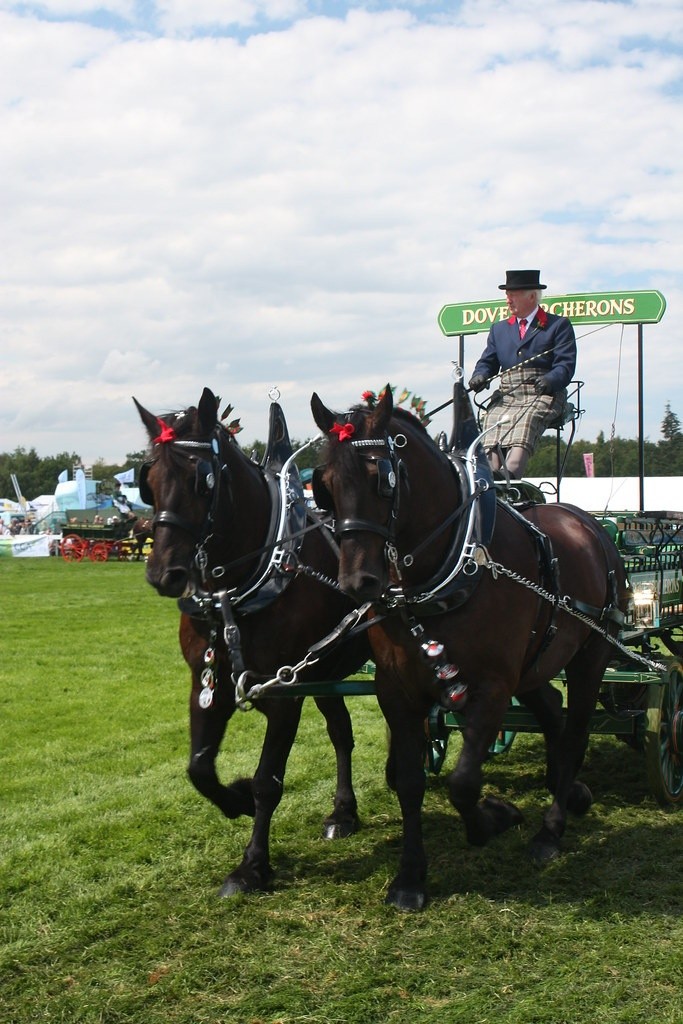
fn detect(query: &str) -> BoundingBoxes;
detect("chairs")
[474,380,588,504]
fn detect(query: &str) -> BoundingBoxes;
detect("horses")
[309,390,632,915]
[132,387,370,903]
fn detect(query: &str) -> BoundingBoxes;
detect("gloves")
[469,374,490,392]
[528,376,552,396]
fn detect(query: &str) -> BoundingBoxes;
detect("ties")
[519,319,528,339]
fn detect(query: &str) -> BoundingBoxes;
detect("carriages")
[60,508,152,562]
[131,380,681,911]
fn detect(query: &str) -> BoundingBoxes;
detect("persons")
[468,269,576,480]
[0,516,34,536]
[111,483,130,516]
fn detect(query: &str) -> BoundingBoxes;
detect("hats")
[498,270,547,289]
